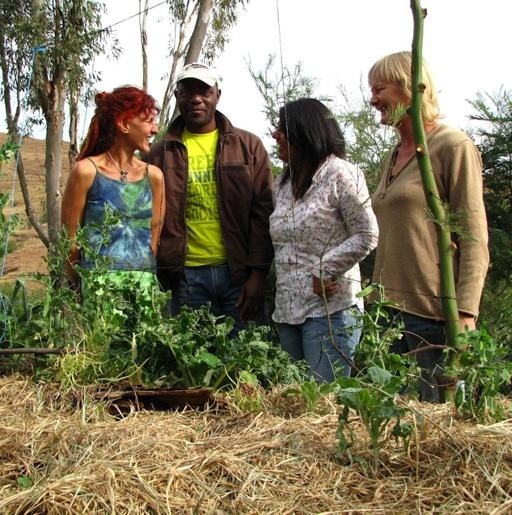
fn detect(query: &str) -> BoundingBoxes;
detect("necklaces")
[107,148,134,184]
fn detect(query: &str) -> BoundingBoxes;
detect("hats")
[170,61,220,88]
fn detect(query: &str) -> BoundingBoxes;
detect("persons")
[60,83,168,319]
[264,96,382,384]
[140,61,275,344]
[366,45,492,406]
